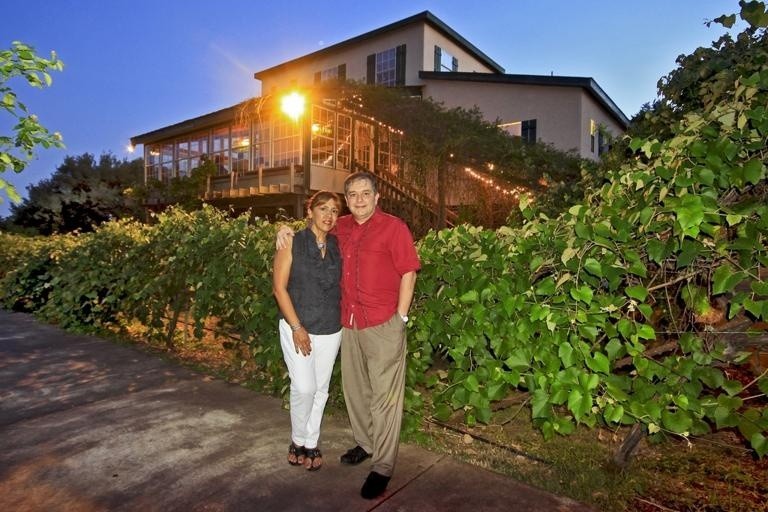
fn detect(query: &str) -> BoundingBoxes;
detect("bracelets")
[290,321,302,332]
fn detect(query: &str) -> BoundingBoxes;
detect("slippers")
[288,442,306,465]
[304,449,323,470]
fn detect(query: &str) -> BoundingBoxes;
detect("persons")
[275,172,421,499]
[272,190,344,472]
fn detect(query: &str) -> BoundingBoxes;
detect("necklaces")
[316,237,326,250]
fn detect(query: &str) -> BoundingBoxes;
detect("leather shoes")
[340,446,372,465]
[361,471,391,499]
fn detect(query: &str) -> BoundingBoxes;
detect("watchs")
[400,315,409,323]
